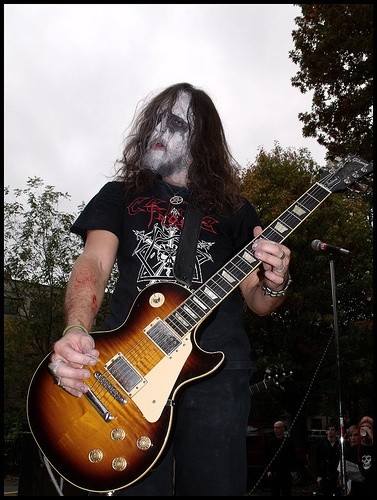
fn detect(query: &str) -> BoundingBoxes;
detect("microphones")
[311,239,350,255]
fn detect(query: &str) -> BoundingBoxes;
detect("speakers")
[17,431,80,496]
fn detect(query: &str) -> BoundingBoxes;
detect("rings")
[56,378,62,386]
[279,251,286,261]
[272,265,285,274]
[48,360,63,378]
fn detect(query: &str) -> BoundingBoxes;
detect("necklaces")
[161,177,186,205]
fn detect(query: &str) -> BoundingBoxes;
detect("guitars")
[27,151,374,493]
[250,366,293,399]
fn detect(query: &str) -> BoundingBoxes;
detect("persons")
[48,83,290,500]
[265,417,373,498]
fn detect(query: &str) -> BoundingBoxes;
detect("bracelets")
[63,322,89,336]
[262,274,292,297]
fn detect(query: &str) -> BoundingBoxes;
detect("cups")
[360,430,368,436]
